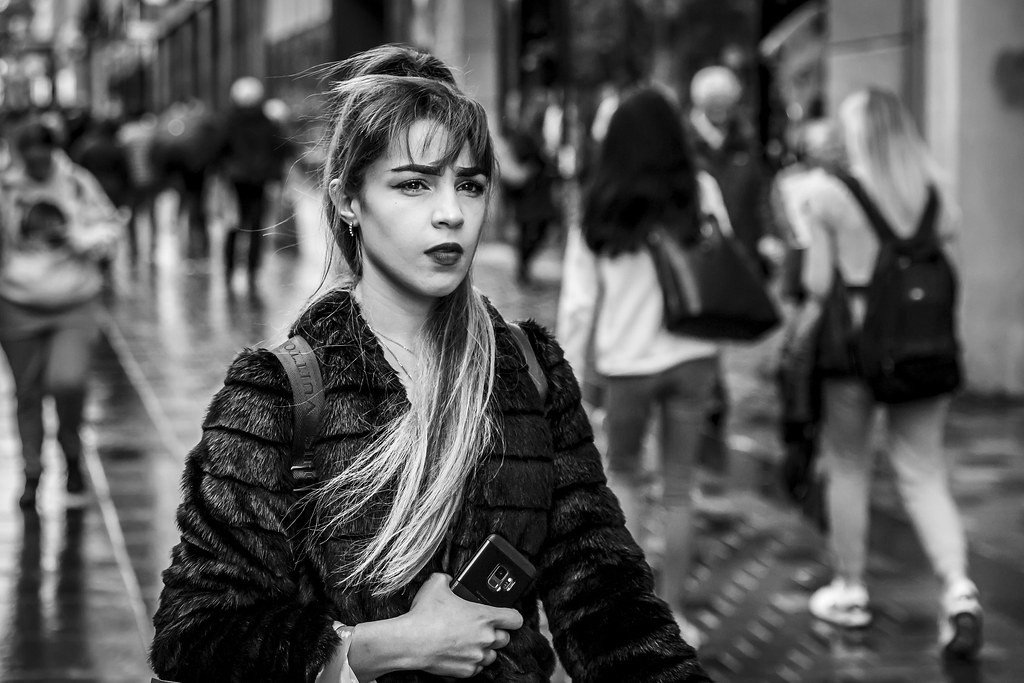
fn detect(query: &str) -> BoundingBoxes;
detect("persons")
[150,45,713,683]
[499,58,986,666]
[2,68,301,509]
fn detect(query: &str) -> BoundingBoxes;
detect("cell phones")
[449,532,540,608]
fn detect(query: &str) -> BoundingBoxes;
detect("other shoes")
[809,581,872,627]
[19,474,40,509]
[66,455,84,493]
[938,586,985,668]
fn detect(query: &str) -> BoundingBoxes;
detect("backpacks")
[823,169,964,408]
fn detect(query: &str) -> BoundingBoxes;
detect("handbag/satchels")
[646,213,784,344]
